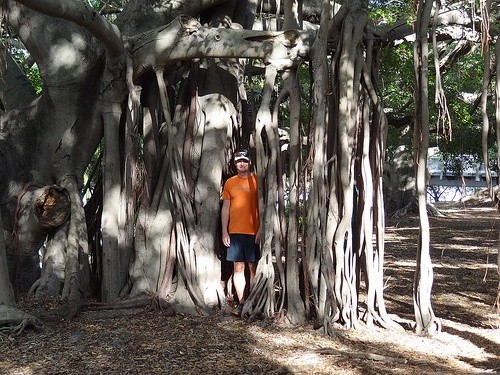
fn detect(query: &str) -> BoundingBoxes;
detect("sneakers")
[232,304,243,316]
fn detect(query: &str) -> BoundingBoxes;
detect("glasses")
[234,153,250,157]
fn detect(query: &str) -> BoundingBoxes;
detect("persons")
[220,147,263,317]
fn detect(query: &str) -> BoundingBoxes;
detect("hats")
[234,148,251,161]
